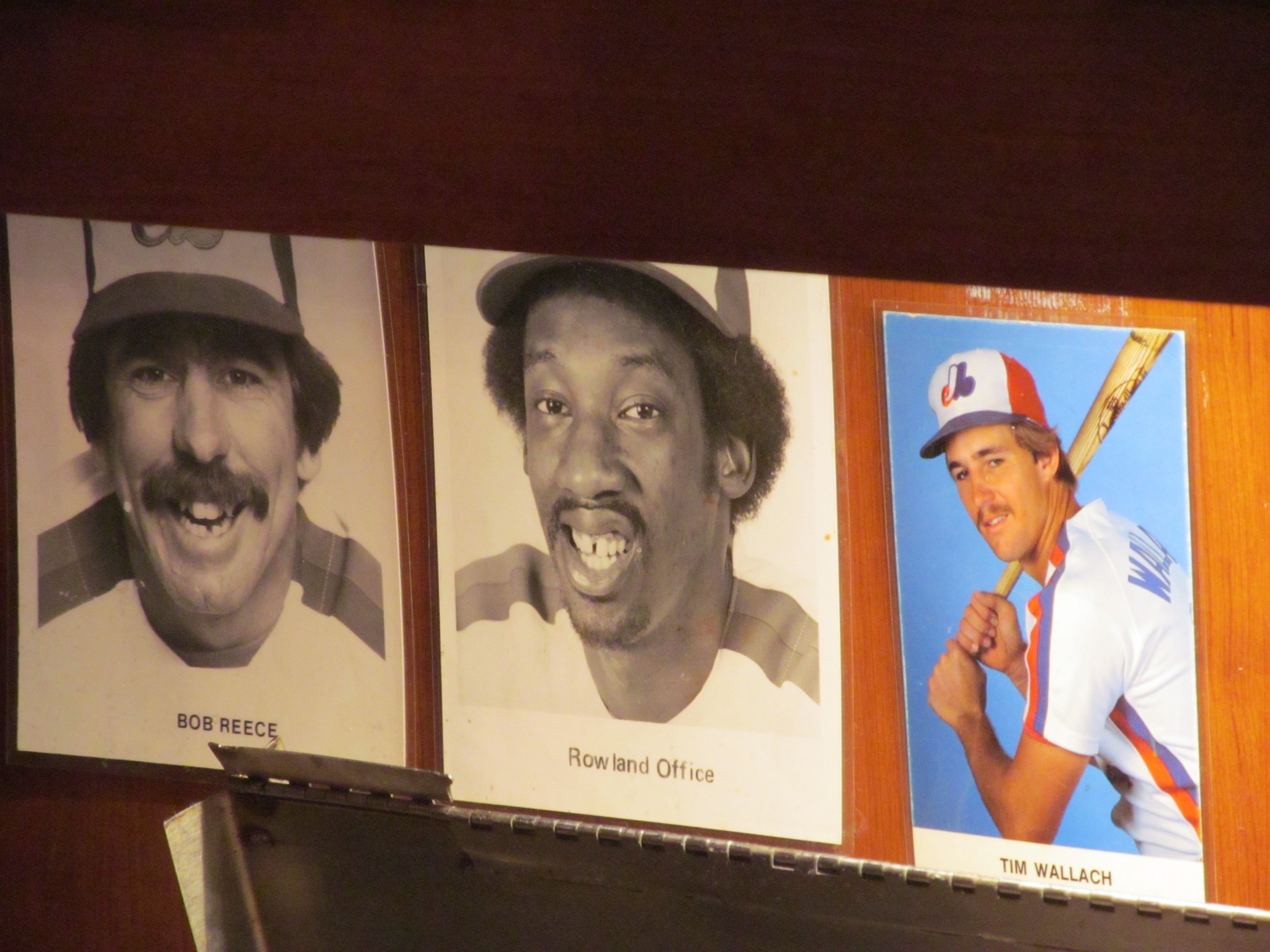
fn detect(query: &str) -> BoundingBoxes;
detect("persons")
[920,348,1203,859]
[36,220,386,670]
[453,253,822,728]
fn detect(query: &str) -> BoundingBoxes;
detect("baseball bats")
[995,330,1175,600]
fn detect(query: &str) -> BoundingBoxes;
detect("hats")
[920,348,1048,459]
[72,219,303,340]
[475,248,752,338]
